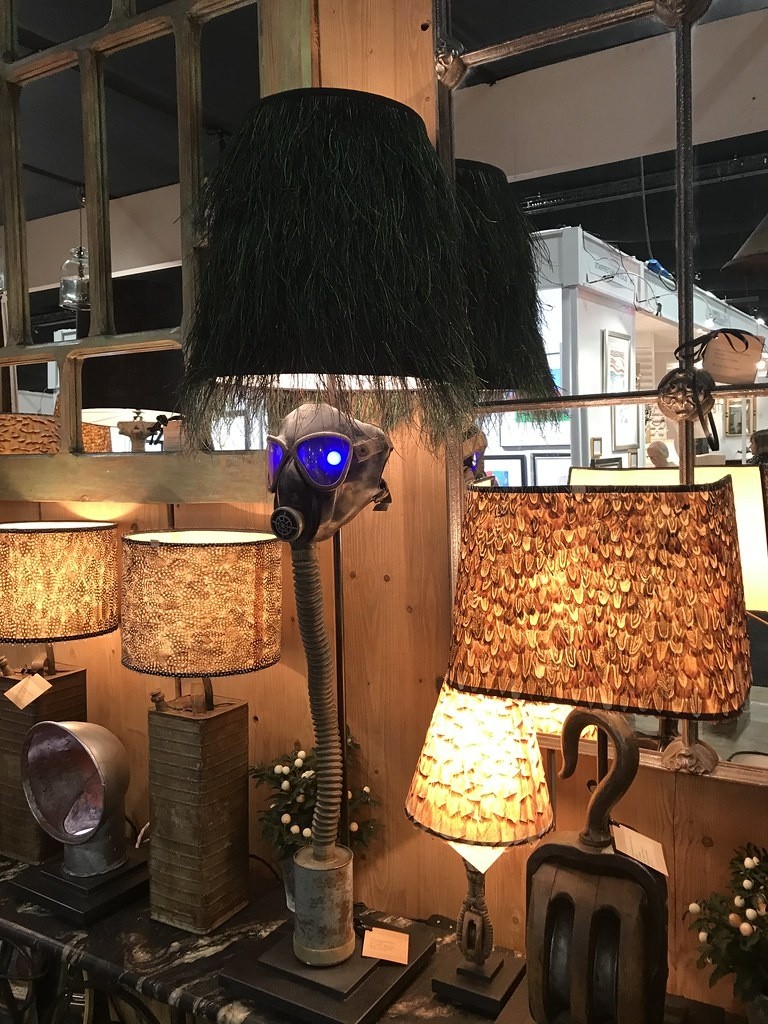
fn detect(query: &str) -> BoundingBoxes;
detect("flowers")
[682,841,768,1005]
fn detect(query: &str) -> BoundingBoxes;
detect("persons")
[646,441,678,467]
[747,429,768,509]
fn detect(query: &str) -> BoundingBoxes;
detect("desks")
[634,686,768,763]
[0,855,748,1024]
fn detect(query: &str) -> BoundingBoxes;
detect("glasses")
[262,434,384,492]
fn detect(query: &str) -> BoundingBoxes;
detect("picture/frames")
[499,352,570,448]
[602,329,632,394]
[590,437,603,458]
[628,449,638,468]
[595,457,622,468]
[611,405,640,452]
[483,455,527,486]
[211,410,251,450]
[530,452,572,486]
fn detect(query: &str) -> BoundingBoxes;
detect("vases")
[283,857,295,913]
[746,994,768,1024]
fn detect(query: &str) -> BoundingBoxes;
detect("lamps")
[179,87,495,1024]
[0,519,120,867]
[0,412,61,455]
[566,464,768,752]
[603,272,662,317]
[120,527,284,935]
[446,475,753,1024]
[521,701,597,741]
[404,672,555,1019]
[660,291,714,327]
[453,157,568,424]
[82,422,112,452]
